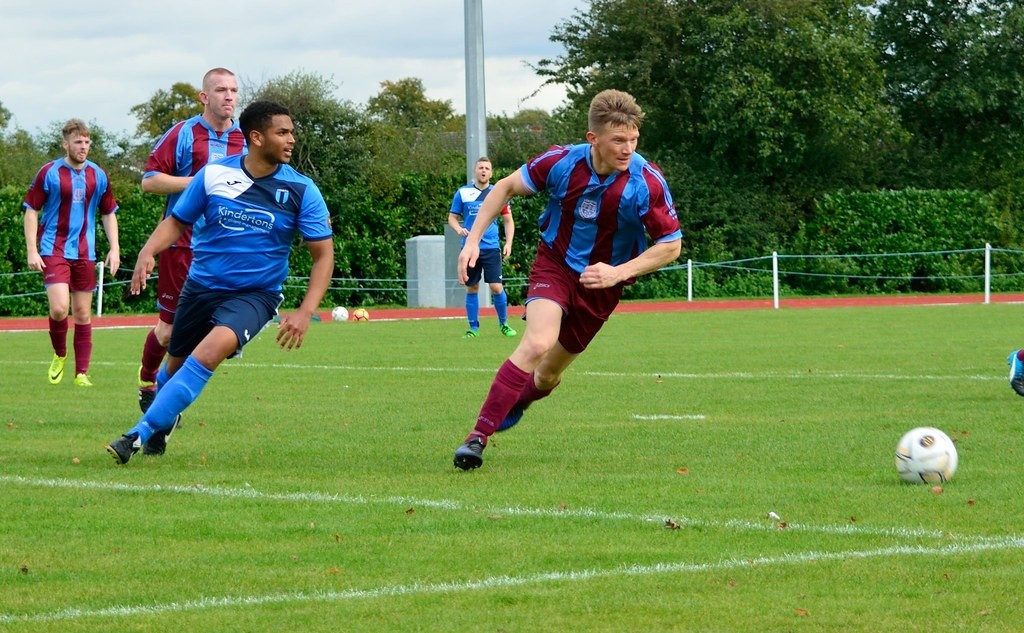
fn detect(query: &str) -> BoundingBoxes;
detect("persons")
[448,158,517,339]
[22,120,120,386]
[1007,349,1024,397]
[453,88,681,470]
[105,102,336,466]
[136,69,251,411]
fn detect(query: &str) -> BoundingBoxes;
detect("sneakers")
[48,352,67,384]
[106,431,142,464]
[499,323,518,337]
[138,387,158,416]
[453,445,484,471]
[73,373,93,389]
[1007,348,1024,396]
[462,329,479,340]
[494,406,524,432]
[143,412,182,455]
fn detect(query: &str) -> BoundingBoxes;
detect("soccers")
[895,426,958,483]
[332,306,349,321]
[352,309,370,321]
